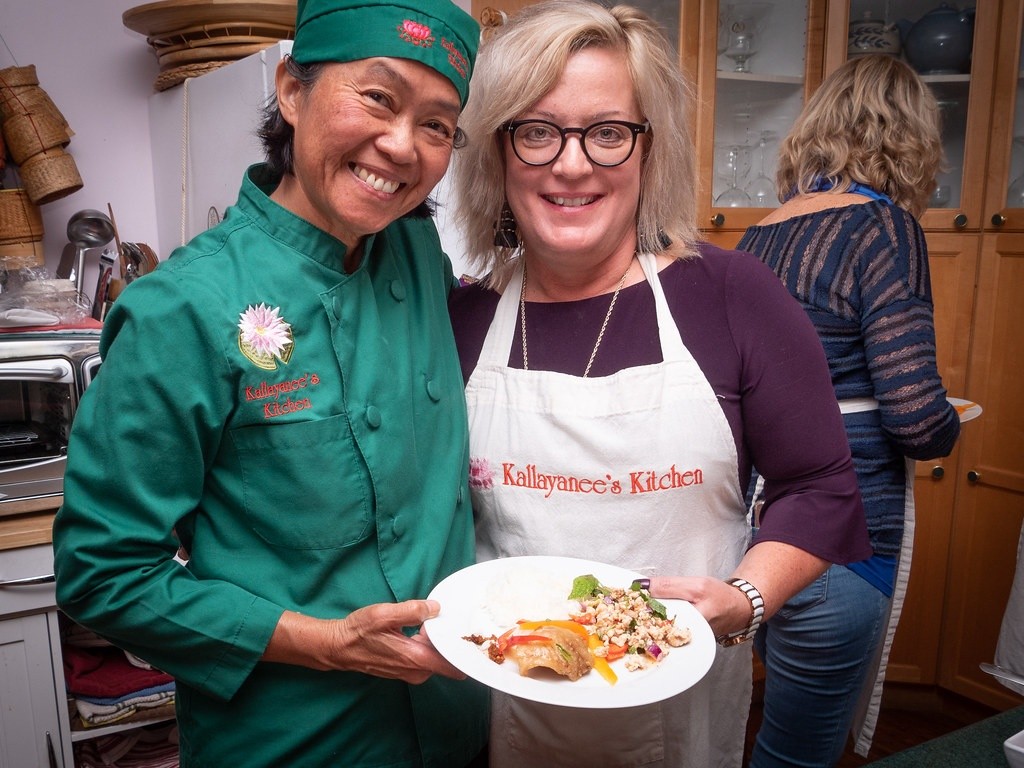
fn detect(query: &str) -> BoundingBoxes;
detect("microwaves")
[0,334,102,499]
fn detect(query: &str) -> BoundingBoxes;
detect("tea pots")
[849,4,975,73]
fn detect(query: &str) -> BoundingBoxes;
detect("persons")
[441,0,873,768]
[726,59,961,768]
[48,0,494,768]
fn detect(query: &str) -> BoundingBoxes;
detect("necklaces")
[521,260,630,377]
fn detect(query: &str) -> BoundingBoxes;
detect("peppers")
[497,621,618,684]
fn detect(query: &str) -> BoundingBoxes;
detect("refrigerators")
[146,39,527,288]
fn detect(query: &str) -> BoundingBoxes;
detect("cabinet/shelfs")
[469,0,1024,710]
[0,532,189,768]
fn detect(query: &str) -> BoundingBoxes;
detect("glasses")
[498,119,650,167]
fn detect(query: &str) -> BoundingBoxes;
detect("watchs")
[715,579,765,648]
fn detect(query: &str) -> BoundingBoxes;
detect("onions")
[579,596,664,662]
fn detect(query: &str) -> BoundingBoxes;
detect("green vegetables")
[567,573,669,654]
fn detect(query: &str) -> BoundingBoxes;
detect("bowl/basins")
[1004,730,1024,768]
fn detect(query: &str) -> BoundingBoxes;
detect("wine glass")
[1007,137,1024,208]
[712,128,777,208]
[716,13,762,74]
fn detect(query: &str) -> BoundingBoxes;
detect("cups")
[929,186,951,208]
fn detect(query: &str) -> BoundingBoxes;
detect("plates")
[424,556,716,709]
[946,397,983,423]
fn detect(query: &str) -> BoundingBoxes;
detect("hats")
[292,0,480,112]
